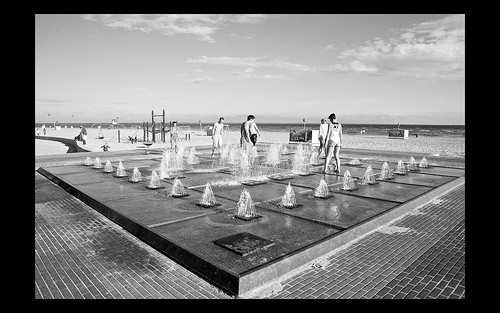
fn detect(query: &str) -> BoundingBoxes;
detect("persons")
[170,120,179,141]
[43,128,46,135]
[80,126,87,145]
[249,122,261,146]
[211,117,224,156]
[322,113,342,174]
[240,115,255,146]
[36,129,39,135]
[319,119,328,156]
[101,142,110,152]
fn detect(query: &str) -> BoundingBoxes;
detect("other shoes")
[321,171,327,174]
[333,169,340,172]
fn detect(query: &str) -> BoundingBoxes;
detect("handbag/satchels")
[250,134,258,143]
